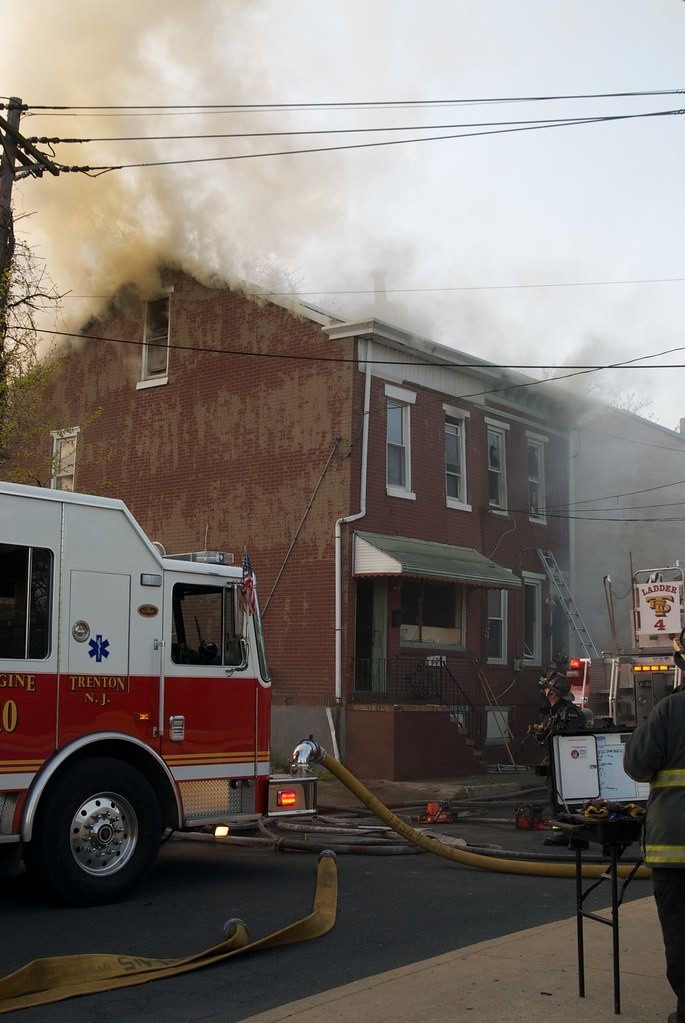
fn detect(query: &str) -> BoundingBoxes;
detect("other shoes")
[668,1012,677,1023]
[567,837,589,851]
[543,831,568,845]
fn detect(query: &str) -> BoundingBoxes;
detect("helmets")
[545,672,575,701]
[673,626,685,672]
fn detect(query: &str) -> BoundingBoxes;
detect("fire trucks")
[535,551,685,821]
[1,479,655,907]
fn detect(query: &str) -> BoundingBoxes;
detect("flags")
[241,551,255,615]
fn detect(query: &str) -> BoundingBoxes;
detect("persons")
[528,672,590,850]
[623,624,685,1022]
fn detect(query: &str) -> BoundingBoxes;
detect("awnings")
[351,531,521,590]
